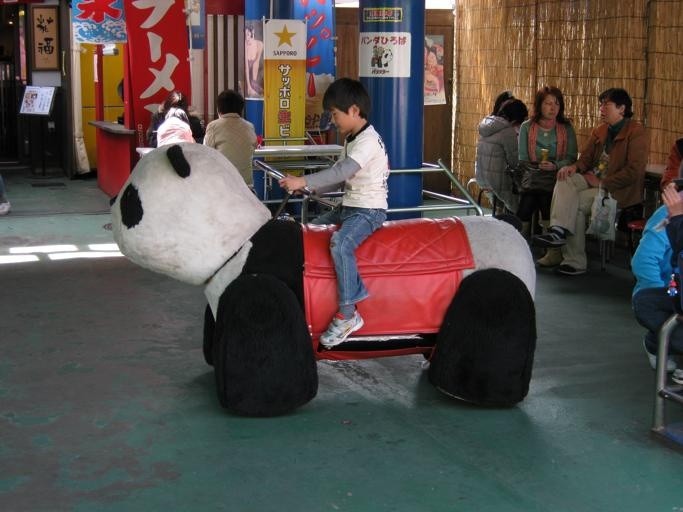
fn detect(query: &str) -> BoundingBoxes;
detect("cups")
[540,148,550,163]
[672,179,683,193]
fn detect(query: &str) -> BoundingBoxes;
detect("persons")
[0,174,11,215]
[244,26,265,99]
[146,88,258,194]
[279,79,391,350]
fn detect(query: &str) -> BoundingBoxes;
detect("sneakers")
[556,264,587,276]
[536,255,558,268]
[536,230,568,247]
[320,308,364,347]
[0,201,11,215]
[642,339,677,373]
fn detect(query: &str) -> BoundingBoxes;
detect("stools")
[477,189,509,218]
[598,203,642,266]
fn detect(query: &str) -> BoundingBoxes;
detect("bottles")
[667,273,678,296]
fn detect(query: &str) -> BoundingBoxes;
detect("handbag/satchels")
[508,159,556,197]
[586,178,617,242]
